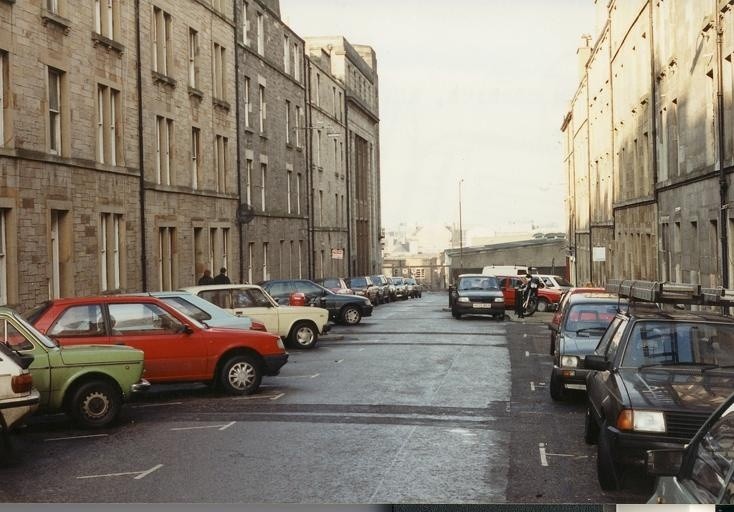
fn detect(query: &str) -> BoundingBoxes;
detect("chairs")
[97,315,122,337]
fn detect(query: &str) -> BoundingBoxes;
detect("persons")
[196,270,214,285]
[209,267,231,285]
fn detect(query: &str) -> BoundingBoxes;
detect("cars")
[2,306,151,430]
[580,312,733,493]
[554,288,607,318]
[179,283,331,350]
[317,274,422,302]
[451,264,572,320]
[64,291,251,331]
[0,342,42,459]
[552,292,665,397]
[639,396,734,504]
[4,294,291,397]
[256,281,373,327]
